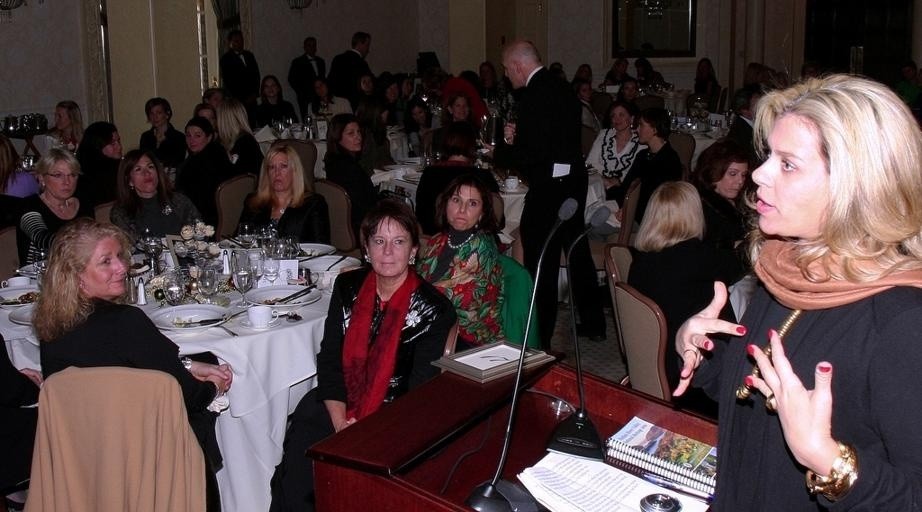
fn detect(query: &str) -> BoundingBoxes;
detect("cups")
[237,223,256,256]
[197,269,218,298]
[32,248,48,277]
[163,278,186,305]
[263,257,280,286]
[279,236,297,259]
[247,258,262,285]
[261,231,279,260]
[1,278,32,289]
[144,236,162,272]
[248,306,279,327]
[298,268,320,286]
[232,267,252,309]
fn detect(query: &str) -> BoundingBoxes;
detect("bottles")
[230,251,239,274]
[128,277,136,304]
[137,278,147,305]
[222,250,230,274]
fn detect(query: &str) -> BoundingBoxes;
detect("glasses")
[41,172,79,181]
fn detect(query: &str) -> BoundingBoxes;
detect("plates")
[0,285,41,308]
[299,255,362,277]
[148,303,233,333]
[8,305,35,326]
[18,264,36,279]
[243,284,323,310]
[299,243,338,257]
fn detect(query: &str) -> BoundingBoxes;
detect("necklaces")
[444,228,478,247]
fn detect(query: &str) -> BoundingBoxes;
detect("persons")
[16,147,88,266]
[34,219,232,474]
[219,100,263,170]
[139,96,188,171]
[630,183,736,376]
[414,139,506,234]
[427,92,486,163]
[615,77,638,103]
[385,78,399,123]
[581,84,603,132]
[178,118,234,228]
[383,81,401,125]
[687,144,751,278]
[686,59,720,114]
[223,33,261,117]
[234,143,329,242]
[258,76,299,127]
[353,73,385,125]
[722,84,763,152]
[571,63,591,97]
[599,58,633,83]
[549,61,566,86]
[0,335,42,500]
[672,75,922,511]
[500,42,606,347]
[0,130,40,220]
[397,80,411,114]
[193,103,219,139]
[585,104,647,189]
[307,76,352,131]
[270,202,455,511]
[327,33,374,101]
[203,89,222,104]
[404,97,440,154]
[476,63,501,99]
[51,100,84,153]
[476,60,499,98]
[411,178,505,351]
[586,107,682,243]
[325,113,390,246]
[110,149,204,243]
[71,121,127,216]
[636,58,667,94]
[289,36,327,123]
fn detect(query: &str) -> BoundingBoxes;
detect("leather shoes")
[573,322,605,341]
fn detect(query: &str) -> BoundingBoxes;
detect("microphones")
[466,198,579,512]
[547,205,611,459]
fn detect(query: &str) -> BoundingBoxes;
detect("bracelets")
[804,444,860,504]
[178,354,193,377]
[203,378,222,396]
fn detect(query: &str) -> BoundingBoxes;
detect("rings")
[765,395,775,412]
[684,348,699,356]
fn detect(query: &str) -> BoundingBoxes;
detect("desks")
[303,340,721,512]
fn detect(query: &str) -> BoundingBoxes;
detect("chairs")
[716,87,729,115]
[602,242,634,360]
[35,365,191,512]
[581,123,599,160]
[668,129,695,184]
[313,178,361,257]
[612,280,671,401]
[376,189,414,213]
[95,201,113,226]
[215,171,256,243]
[498,253,538,349]
[440,318,461,374]
[433,192,506,236]
[0,221,21,288]
[584,179,644,264]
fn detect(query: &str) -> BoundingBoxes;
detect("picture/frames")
[429,338,557,383]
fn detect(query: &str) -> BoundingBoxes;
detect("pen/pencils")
[639,468,715,505]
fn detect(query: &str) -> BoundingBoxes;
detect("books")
[515,453,709,512]
[608,416,718,496]
